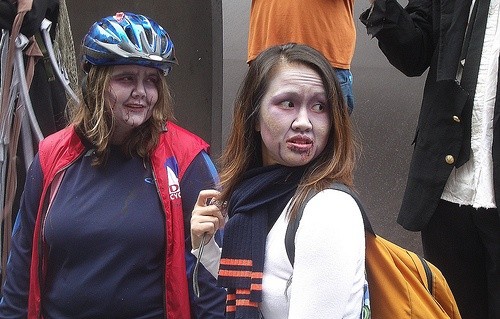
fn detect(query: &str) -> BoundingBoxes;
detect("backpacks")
[284,180,461,319]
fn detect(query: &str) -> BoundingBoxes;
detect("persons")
[246,0,357,116]
[359,0,500,319]
[190,42,372,319]
[0,11,229,319]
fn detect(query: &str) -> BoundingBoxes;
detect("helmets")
[81,12,179,75]
